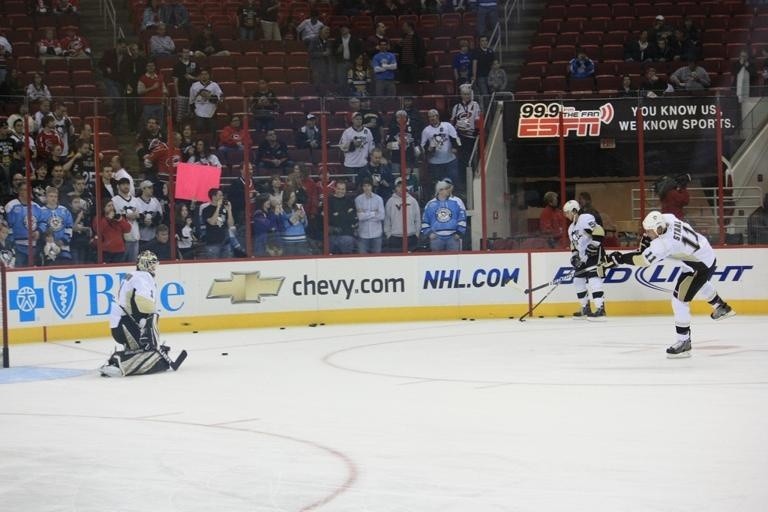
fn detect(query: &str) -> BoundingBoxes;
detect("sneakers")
[666,339,692,354]
[710,302,731,320]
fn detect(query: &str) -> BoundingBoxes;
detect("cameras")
[222,200,228,205]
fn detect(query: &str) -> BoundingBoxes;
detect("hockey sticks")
[505,261,617,294]
[519,284,560,322]
[113,299,187,370]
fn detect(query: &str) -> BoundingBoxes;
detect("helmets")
[136,249,159,276]
[563,200,580,213]
[642,211,666,230]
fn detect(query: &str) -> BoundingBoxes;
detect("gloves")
[574,261,585,271]
[570,254,580,265]
[606,251,624,267]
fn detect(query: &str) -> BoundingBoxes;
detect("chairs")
[514,1,768,99]
[509,230,630,251]
[0,0,509,253]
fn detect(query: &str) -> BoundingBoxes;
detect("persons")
[539,191,603,249]
[619,10,758,95]
[561,199,607,322]
[607,210,736,354]
[1,0,498,267]
[658,175,690,220]
[99,250,172,378]
[569,51,595,78]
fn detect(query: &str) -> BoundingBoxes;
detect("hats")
[396,110,408,116]
[428,109,439,116]
[364,113,378,119]
[141,180,154,191]
[394,177,403,185]
[351,112,362,119]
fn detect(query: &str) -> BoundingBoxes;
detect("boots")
[587,302,606,317]
[574,300,591,316]
